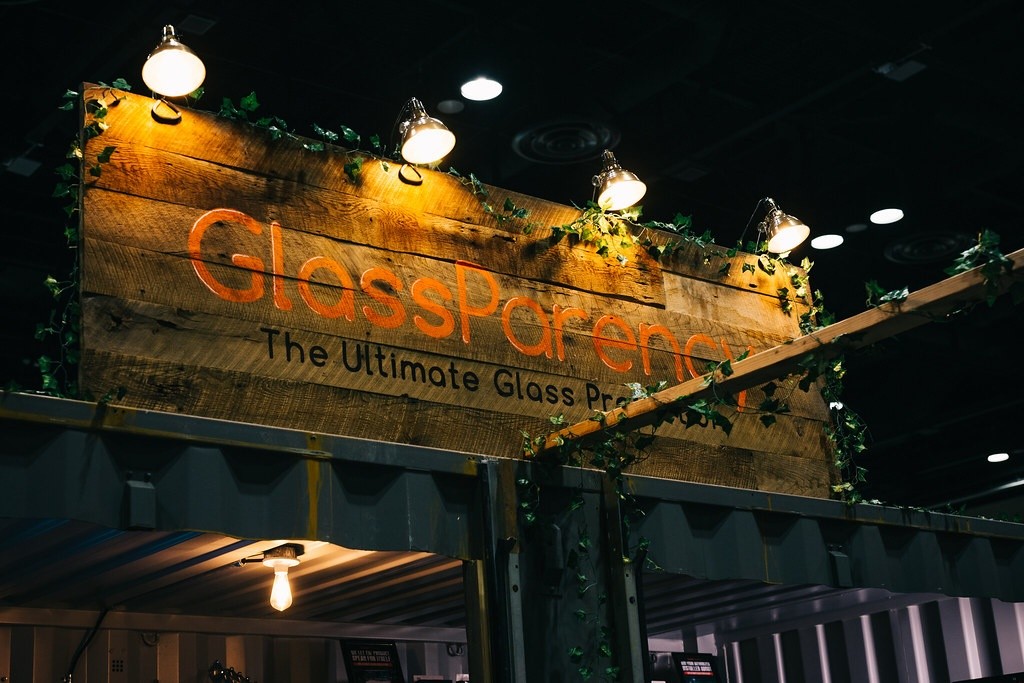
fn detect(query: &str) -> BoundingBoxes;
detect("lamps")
[398,97,455,186]
[261,548,302,613]
[588,150,647,234]
[739,197,812,275]
[140,24,210,122]
